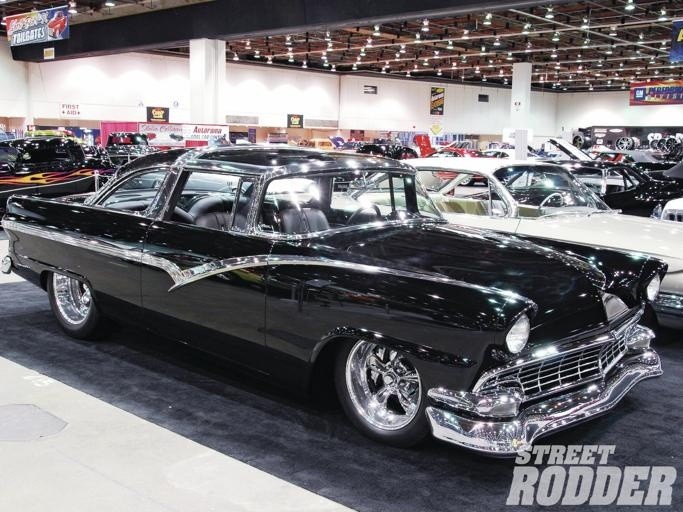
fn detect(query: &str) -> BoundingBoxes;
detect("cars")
[0,138,683,459]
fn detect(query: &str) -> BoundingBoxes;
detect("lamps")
[188,192,331,236]
[431,193,515,215]
